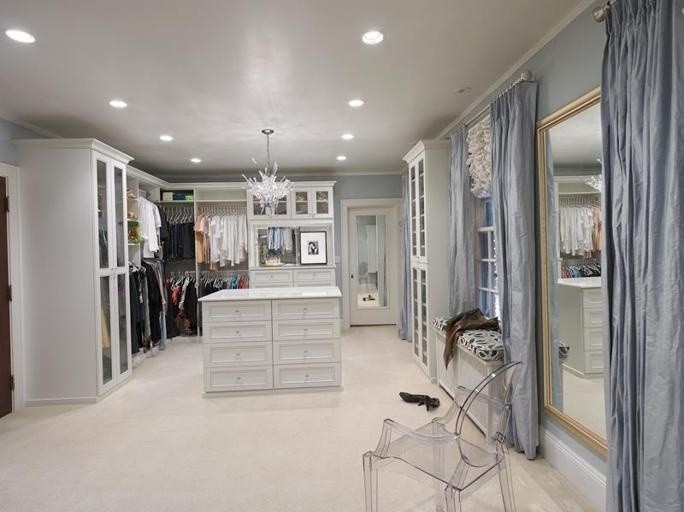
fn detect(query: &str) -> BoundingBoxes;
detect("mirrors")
[353,214,390,311]
[534,85,609,464]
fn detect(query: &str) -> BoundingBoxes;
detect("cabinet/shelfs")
[167,182,335,343]
[557,276,605,380]
[402,138,452,379]
[202,298,342,393]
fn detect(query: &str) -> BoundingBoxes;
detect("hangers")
[557,195,602,270]
[160,203,246,226]
[128,255,250,288]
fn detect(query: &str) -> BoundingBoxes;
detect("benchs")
[432,313,505,443]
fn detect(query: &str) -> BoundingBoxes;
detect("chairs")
[358,262,369,293]
[363,361,526,512]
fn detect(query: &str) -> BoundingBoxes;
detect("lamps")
[240,128,294,218]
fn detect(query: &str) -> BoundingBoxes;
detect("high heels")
[399,392,439,411]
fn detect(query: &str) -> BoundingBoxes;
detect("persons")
[309,242,318,254]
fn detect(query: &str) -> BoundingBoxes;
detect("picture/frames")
[299,231,328,266]
